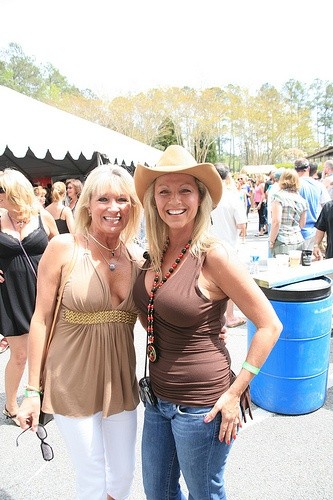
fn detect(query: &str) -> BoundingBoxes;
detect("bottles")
[302,249,312,266]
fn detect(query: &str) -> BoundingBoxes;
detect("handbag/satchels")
[139,376,156,406]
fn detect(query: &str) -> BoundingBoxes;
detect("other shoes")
[239,225,267,238]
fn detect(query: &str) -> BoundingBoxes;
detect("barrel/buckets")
[248,272,333,416]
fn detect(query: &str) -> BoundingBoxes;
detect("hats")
[134,144,222,210]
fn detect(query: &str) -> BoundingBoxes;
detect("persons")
[17,164,228,500]
[33,186,47,206]
[44,181,76,234]
[231,158,333,238]
[132,144,283,500]
[293,157,332,251]
[211,162,248,329]
[313,199,333,262]
[0,167,61,427]
[0,337,10,353]
[64,179,84,219]
[268,168,309,262]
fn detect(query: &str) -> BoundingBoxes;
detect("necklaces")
[85,226,121,256]
[91,238,122,271]
[146,236,193,363]
[8,211,27,230]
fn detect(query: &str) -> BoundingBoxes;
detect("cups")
[289,250,302,266]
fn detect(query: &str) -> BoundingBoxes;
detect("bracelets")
[270,240,275,245]
[242,361,260,376]
[314,244,319,248]
[23,385,44,398]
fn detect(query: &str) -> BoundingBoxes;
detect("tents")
[0,84,165,183]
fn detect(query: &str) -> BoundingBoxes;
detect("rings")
[233,417,240,425]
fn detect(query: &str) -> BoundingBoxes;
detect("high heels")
[2,404,20,427]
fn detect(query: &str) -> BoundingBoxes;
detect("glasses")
[237,178,243,180]
[16,423,54,462]
[0,187,6,194]
